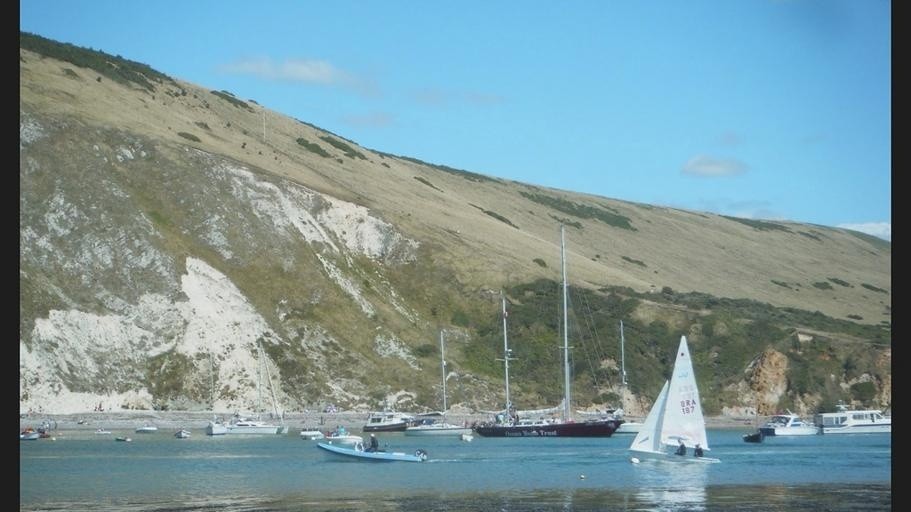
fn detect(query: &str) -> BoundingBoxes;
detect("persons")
[363,434,379,453]
[673,438,686,456]
[693,443,703,458]
[335,425,339,436]
[339,425,345,435]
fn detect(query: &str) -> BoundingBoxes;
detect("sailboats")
[629,335,721,467]
[227,342,289,433]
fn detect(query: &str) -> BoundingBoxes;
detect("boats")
[20,426,190,442]
[744,410,891,443]
[299,430,428,463]
[206,422,225,435]
